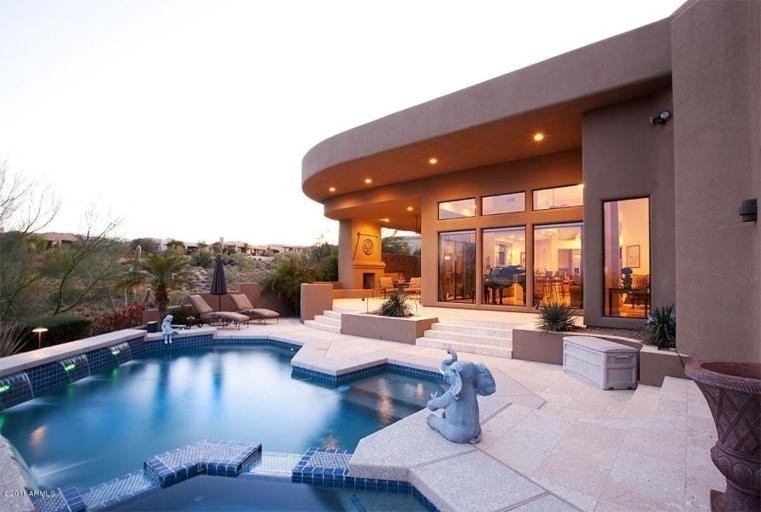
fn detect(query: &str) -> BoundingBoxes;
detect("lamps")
[31,325,48,349]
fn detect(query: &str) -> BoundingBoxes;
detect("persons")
[160,314,174,345]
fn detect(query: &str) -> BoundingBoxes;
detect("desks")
[608,287,650,318]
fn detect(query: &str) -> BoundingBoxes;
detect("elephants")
[426,346,497,445]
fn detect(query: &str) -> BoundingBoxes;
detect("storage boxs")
[562,335,639,390]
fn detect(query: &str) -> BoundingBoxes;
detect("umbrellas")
[209,253,229,311]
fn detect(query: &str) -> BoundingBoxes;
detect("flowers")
[619,266,634,285]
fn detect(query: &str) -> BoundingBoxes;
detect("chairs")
[535,270,574,300]
[187,293,280,331]
[373,276,427,298]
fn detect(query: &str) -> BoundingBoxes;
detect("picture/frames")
[625,244,641,270]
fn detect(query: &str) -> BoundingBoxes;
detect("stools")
[472,289,489,305]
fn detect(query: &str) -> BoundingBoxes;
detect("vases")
[624,283,632,292]
